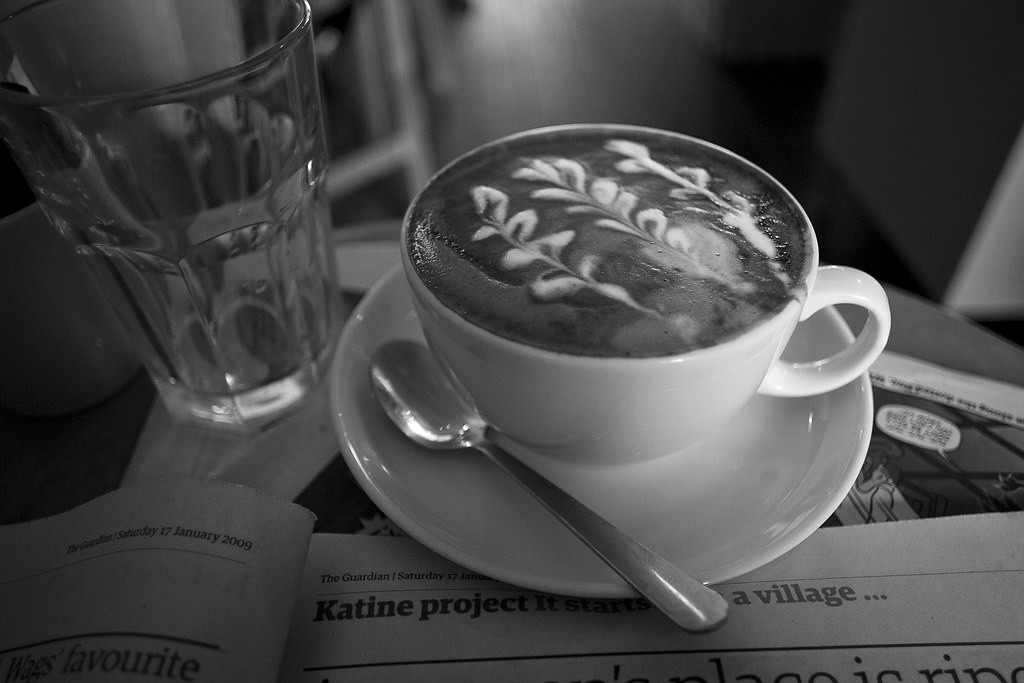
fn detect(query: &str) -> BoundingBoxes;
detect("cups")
[398,122,893,471]
[1,0,346,438]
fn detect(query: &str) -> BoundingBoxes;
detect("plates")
[328,259,876,599]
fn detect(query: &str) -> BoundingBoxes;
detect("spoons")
[370,338,728,635]
